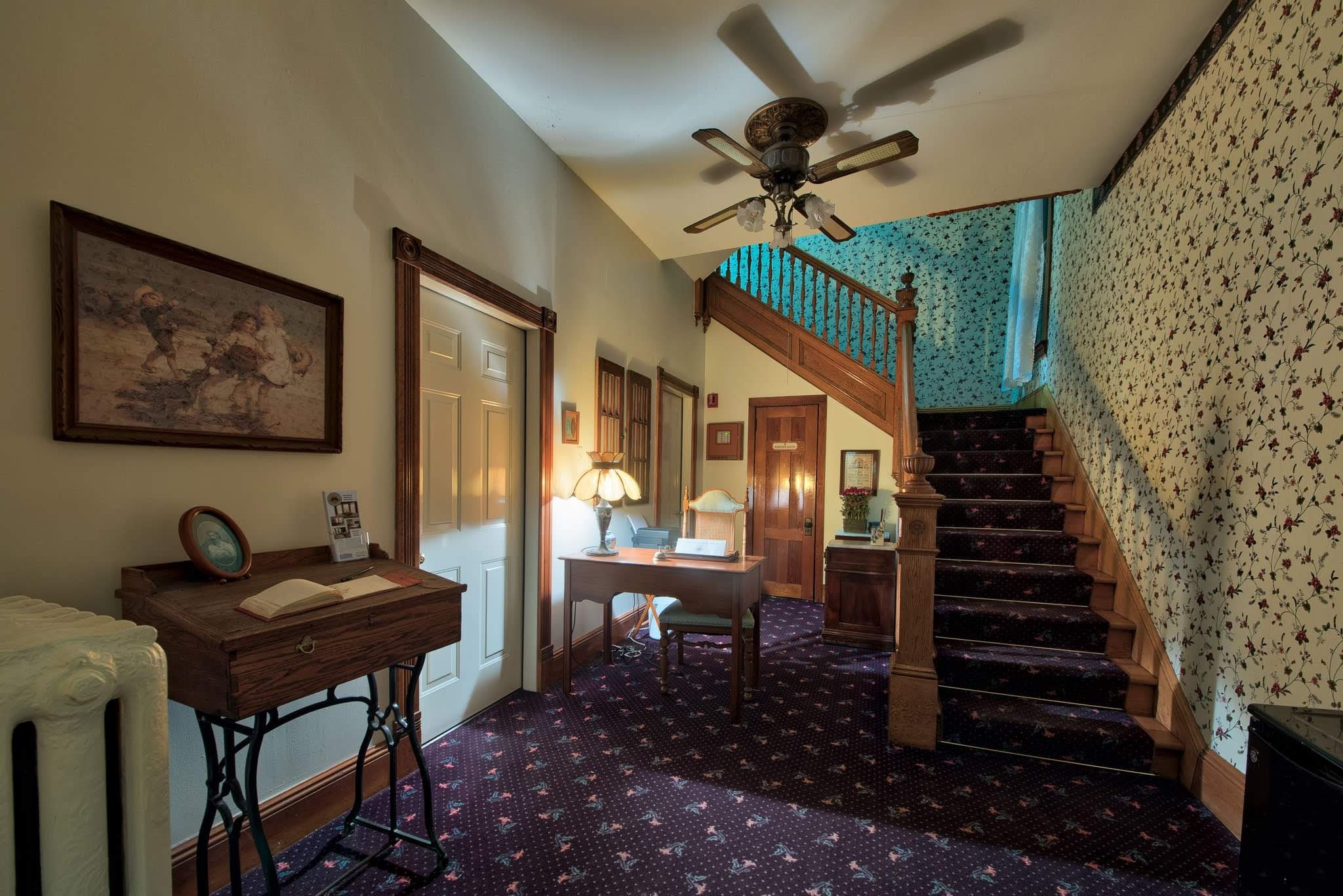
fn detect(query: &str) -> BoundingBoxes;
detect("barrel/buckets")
[648,596,677,641]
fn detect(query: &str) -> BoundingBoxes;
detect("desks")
[557,547,769,704]
[112,543,468,896]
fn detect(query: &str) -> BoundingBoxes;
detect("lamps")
[737,182,835,250]
[568,452,642,556]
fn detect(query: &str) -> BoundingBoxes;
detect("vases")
[843,518,868,533]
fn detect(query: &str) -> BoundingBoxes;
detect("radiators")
[0,595,175,896]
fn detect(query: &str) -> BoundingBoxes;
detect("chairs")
[627,514,659,638]
[658,485,755,701]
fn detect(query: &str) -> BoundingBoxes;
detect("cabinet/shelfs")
[821,539,896,651]
[1236,704,1343,896]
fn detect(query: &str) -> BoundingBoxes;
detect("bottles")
[868,522,879,533]
[605,530,616,548]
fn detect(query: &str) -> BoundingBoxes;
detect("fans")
[683,96,919,243]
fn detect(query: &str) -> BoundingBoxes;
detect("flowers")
[839,487,873,519]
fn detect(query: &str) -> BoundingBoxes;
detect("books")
[234,572,402,621]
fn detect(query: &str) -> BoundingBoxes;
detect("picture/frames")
[562,409,580,445]
[48,200,345,453]
[179,506,253,584]
[839,450,879,496]
